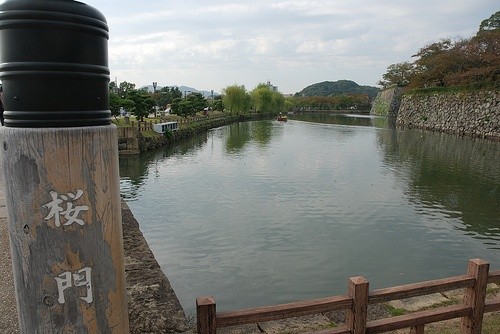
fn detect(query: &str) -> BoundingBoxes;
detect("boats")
[277,115,287,121]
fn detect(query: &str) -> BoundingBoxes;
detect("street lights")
[153,82,157,118]
[185,91,191,100]
